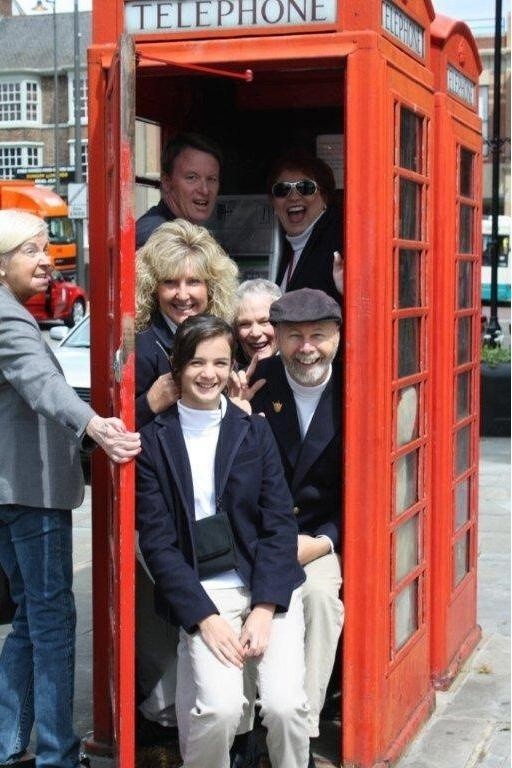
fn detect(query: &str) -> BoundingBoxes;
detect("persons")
[128,220,344,768]
[269,154,346,368]
[133,133,230,255]
[0,209,142,768]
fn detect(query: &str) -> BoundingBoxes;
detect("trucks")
[1,179,79,275]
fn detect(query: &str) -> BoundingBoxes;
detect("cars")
[24,270,87,327]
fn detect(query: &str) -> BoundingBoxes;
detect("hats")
[267,288,343,324]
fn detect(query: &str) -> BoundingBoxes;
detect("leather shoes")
[0,758,36,768]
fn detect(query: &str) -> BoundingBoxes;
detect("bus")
[479,212,511,305]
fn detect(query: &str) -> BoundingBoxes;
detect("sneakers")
[137,706,178,746]
[232,749,261,767]
[322,681,342,720]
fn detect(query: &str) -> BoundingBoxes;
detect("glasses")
[271,179,321,198]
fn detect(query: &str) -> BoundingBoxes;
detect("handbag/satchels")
[193,511,237,578]
